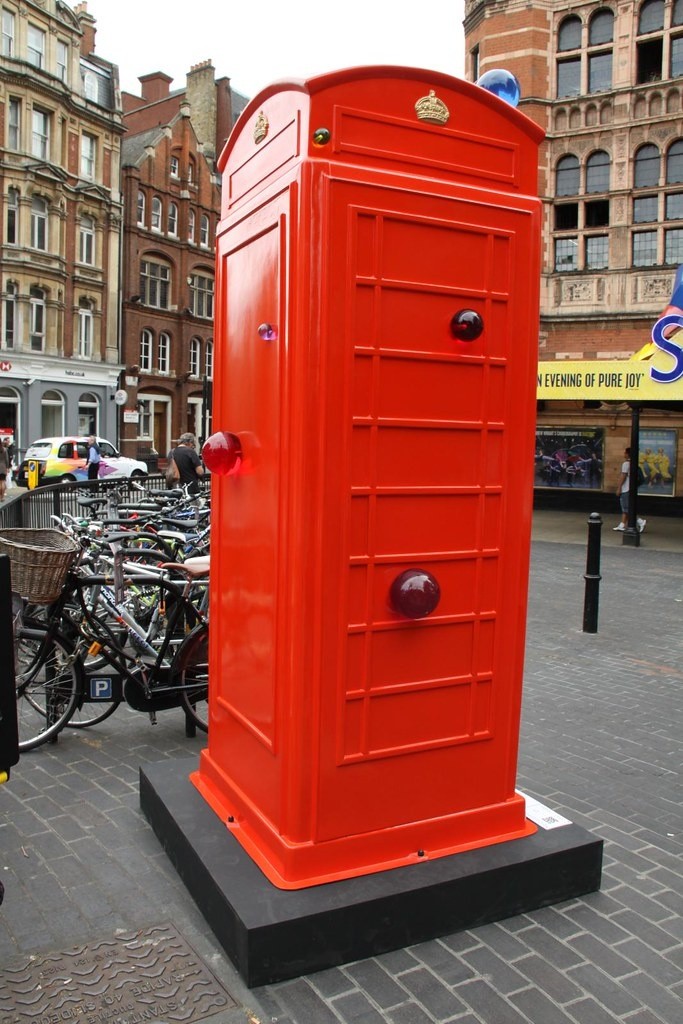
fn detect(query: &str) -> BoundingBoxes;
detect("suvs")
[16,434,150,493]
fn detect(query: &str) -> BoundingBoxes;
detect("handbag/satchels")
[165,448,180,489]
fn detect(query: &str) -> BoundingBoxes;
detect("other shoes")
[638,520,646,533]
[613,526,627,532]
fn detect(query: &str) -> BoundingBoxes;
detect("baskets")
[0,527,81,607]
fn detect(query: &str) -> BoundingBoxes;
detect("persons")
[166,439,195,490]
[0,438,9,502]
[638,448,671,488]
[82,435,100,494]
[174,433,204,494]
[3,437,14,489]
[612,447,646,532]
[535,448,601,489]
[100,447,107,458]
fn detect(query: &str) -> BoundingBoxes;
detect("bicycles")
[0,478,209,754]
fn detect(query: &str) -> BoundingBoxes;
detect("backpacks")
[625,460,645,488]
